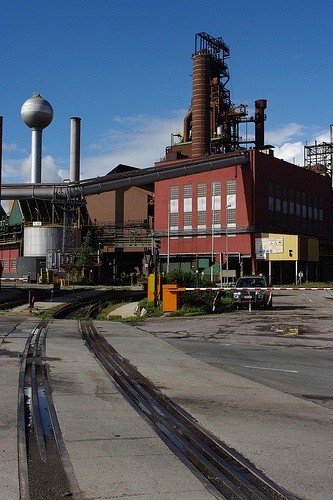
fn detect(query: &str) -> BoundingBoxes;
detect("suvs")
[231,274,272,310]
